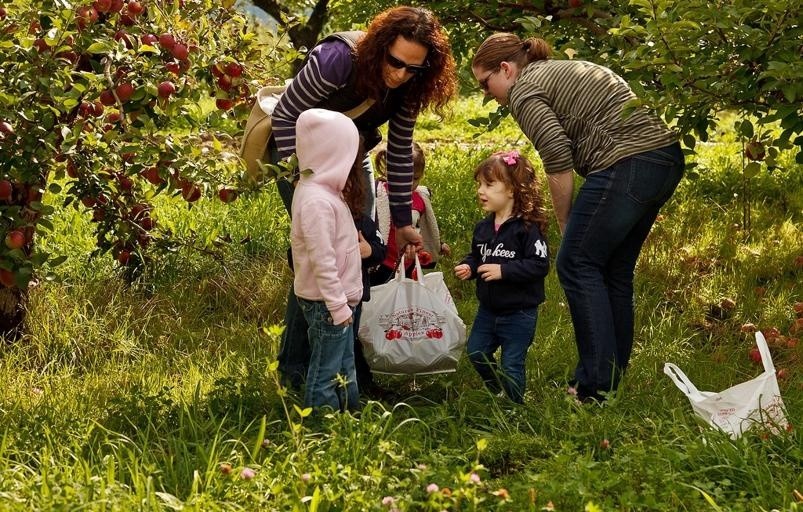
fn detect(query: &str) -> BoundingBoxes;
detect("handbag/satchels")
[240,80,289,181]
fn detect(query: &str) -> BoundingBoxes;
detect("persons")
[289,108,365,419]
[452,152,550,406]
[375,142,450,286]
[269,6,456,404]
[472,32,687,413]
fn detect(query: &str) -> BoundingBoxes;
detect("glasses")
[479,60,505,91]
[385,53,426,74]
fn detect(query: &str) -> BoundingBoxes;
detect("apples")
[418,252,432,266]
[643,74,802,382]
[384,329,401,340]
[0,0,261,287]
[426,328,442,338]
